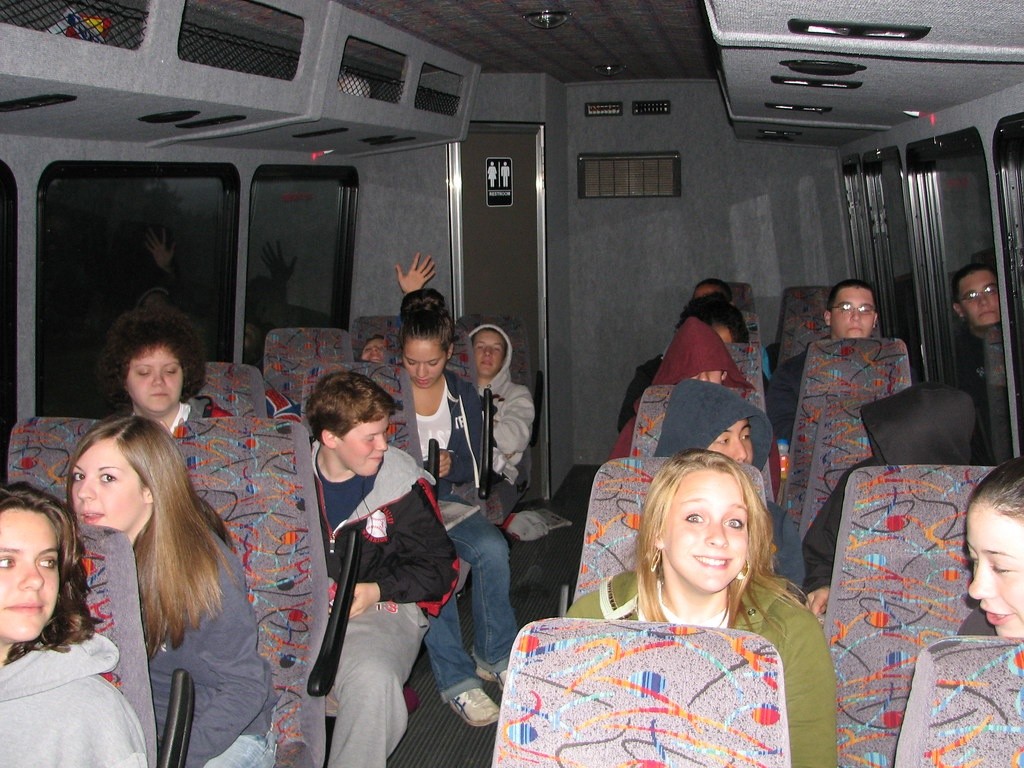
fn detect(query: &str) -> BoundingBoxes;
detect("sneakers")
[450,688,499,728]
[475,663,508,692]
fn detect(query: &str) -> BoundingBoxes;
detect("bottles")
[776,439,789,480]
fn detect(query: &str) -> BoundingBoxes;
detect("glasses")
[959,285,998,301]
[831,303,875,315]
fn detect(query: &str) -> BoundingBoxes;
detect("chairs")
[5,277,1024,768]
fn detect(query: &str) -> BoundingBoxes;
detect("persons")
[950,265,1013,460]
[144,225,186,303]
[566,277,1023,768]
[1,254,534,768]
